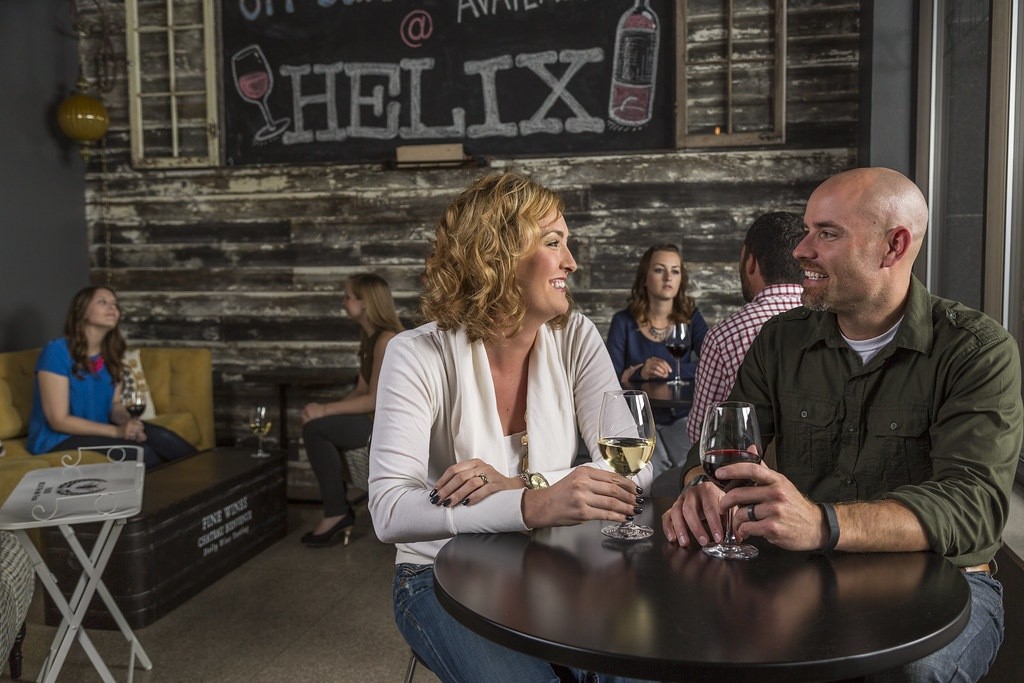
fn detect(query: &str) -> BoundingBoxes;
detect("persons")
[301,274,405,546]
[663,166,1024,683]
[607,242,707,473]
[27,286,197,468]
[367,173,654,683]
[689,212,810,447]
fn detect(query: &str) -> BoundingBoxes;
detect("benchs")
[0,347,215,507]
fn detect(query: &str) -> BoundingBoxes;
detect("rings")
[747,505,756,521]
[630,366,633,369]
[480,475,488,484]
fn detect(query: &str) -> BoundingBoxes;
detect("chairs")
[341,447,369,504]
[0,530,35,683]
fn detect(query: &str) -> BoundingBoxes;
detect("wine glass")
[664,321,690,387]
[250,406,273,458]
[597,390,657,541]
[125,390,147,420]
[699,401,762,560]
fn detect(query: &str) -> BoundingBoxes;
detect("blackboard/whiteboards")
[215,0,686,174]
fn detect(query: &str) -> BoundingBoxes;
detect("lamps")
[56,25,109,164]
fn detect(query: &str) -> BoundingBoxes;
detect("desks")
[41,446,288,629]
[621,378,694,407]
[432,497,972,683]
[0,445,153,682]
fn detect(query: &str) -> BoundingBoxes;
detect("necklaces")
[521,411,528,471]
[649,320,667,340]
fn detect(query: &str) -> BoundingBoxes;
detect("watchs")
[819,503,840,552]
[514,472,549,489]
[687,475,723,489]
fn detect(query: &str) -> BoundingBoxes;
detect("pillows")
[121,349,156,420]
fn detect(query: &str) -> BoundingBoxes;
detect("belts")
[960,562,990,575]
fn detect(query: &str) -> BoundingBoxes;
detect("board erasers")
[396,144,464,167]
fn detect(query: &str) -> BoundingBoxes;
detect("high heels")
[301,505,356,545]
